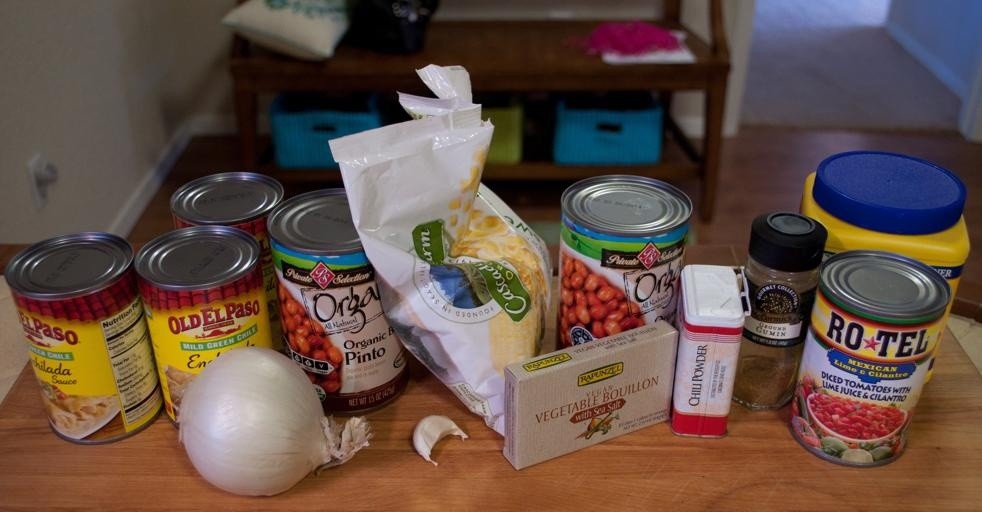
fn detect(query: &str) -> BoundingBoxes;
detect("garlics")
[176,346,373,495]
[413,415,470,466]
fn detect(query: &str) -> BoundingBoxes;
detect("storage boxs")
[269,89,383,168]
[479,102,525,167]
[551,89,666,165]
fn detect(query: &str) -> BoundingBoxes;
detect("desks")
[0,243,977,512]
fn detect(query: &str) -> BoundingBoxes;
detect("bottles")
[732,214,829,412]
[796,148,974,388]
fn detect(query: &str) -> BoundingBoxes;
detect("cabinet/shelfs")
[228,0,732,222]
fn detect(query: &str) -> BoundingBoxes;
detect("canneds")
[3,168,417,445]
[787,248,953,468]
[554,174,693,353]
